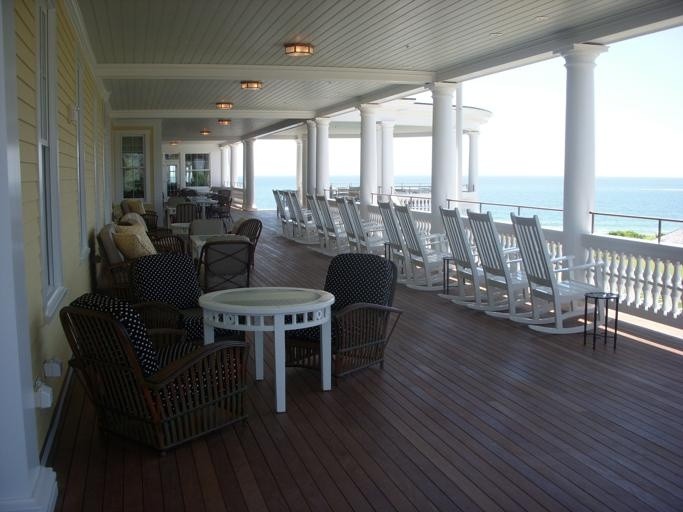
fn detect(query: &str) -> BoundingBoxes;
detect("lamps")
[199,42,314,137]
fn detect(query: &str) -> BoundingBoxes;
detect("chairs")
[437,204,516,307]
[333,195,385,255]
[307,193,347,254]
[285,253,404,378]
[376,198,450,293]
[272,188,320,247]
[90,185,266,293]
[465,207,552,319]
[59,291,251,454]
[505,210,604,335]
[110,253,235,339]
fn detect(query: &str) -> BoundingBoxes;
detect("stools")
[582,292,620,352]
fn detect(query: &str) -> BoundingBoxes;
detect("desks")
[198,285,336,413]
